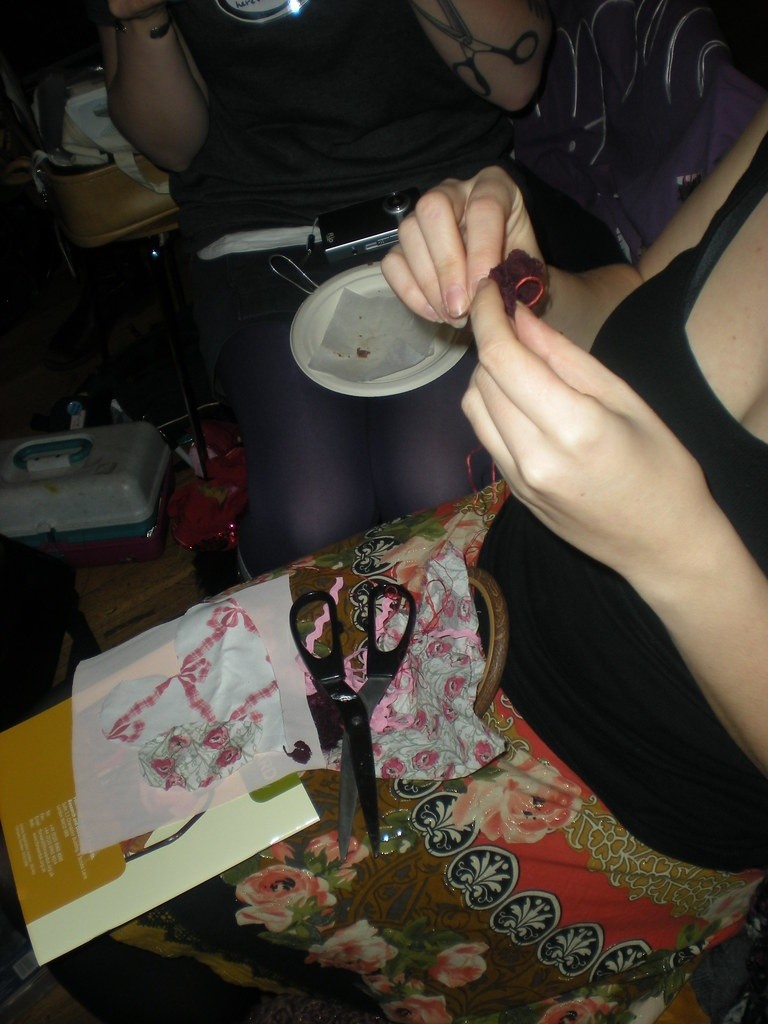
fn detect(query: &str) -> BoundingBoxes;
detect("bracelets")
[112,14,172,39]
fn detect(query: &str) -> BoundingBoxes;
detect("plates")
[290,261,477,400]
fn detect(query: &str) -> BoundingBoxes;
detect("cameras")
[317,186,421,264]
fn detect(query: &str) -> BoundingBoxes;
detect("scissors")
[288,583,421,866]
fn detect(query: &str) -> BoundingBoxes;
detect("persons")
[88,1,549,586]
[1,89,767,1023]
